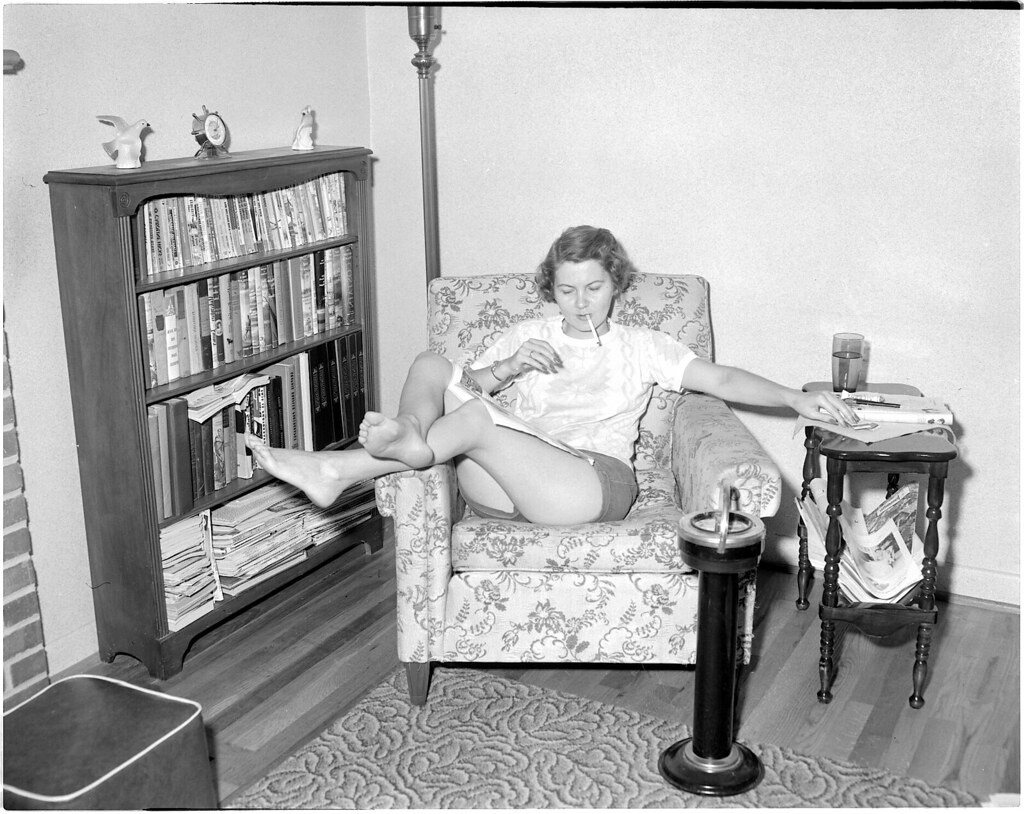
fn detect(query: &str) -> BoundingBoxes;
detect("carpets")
[218,662,981,809]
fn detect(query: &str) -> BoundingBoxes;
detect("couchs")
[374,272,782,706]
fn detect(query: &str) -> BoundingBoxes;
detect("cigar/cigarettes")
[587,315,600,344]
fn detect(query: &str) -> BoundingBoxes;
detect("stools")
[3,674,219,810]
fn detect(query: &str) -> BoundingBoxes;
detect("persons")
[241,225,859,526]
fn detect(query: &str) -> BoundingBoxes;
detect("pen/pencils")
[844,397,901,409]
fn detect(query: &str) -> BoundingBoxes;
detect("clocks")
[190,104,232,159]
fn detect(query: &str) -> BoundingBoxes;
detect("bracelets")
[491,361,503,381]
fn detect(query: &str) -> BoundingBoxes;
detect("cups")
[831,332,864,393]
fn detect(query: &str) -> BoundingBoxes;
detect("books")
[794,392,954,604]
[448,359,594,463]
[131,173,376,632]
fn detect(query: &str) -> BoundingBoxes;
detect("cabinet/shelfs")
[42,144,383,680]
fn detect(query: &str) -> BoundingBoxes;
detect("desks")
[795,380,956,708]
